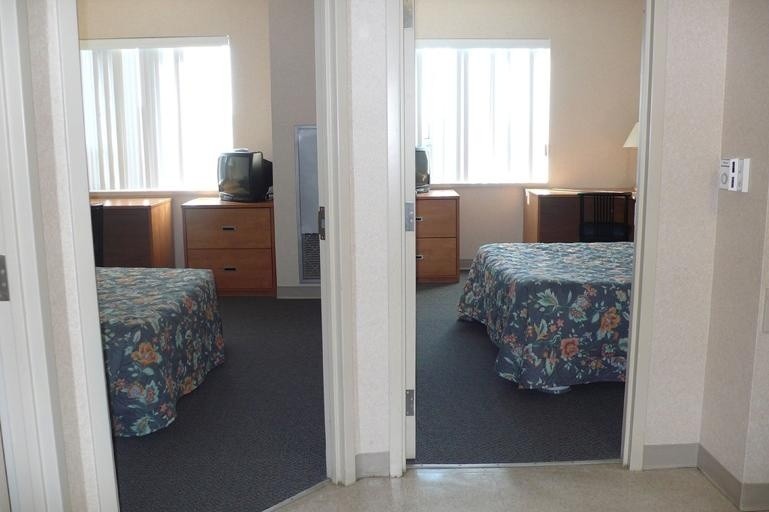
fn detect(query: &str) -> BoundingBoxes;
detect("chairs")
[578,191,632,242]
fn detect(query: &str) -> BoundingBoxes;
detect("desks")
[523,187,637,246]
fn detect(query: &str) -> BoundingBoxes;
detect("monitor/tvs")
[415,148,430,193]
[217,151,273,201]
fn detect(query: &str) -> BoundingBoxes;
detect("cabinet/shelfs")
[89,198,173,270]
[181,196,274,299]
[415,190,462,285]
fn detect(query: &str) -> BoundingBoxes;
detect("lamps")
[622,121,640,148]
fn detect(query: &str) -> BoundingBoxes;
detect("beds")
[475,241,636,391]
[94,266,224,438]
